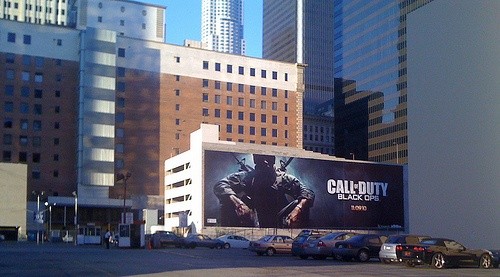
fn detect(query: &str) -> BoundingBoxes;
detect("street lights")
[31,190,57,245]
[117,172,131,223]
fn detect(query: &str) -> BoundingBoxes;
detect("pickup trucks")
[175,234,225,249]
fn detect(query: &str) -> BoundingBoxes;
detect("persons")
[104,229,110,249]
[212,154,315,229]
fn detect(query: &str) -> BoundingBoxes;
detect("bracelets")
[296,205,304,213]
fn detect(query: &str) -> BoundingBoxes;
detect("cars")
[307,231,360,260]
[215,235,251,249]
[145,231,184,248]
[62,234,74,243]
[109,233,118,244]
[378,235,431,265]
[333,234,389,262]
[248,235,295,256]
[291,230,329,259]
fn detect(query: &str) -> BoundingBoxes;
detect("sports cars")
[396,238,495,269]
